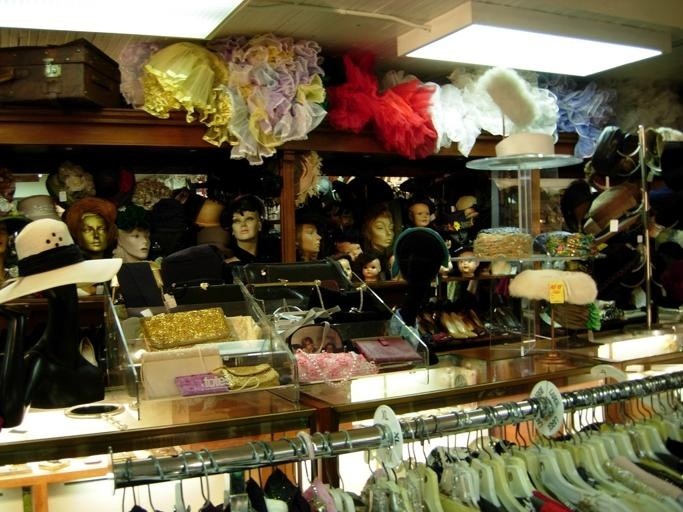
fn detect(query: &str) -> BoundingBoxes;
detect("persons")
[557,178,637,311]
[325,342,336,353]
[17,283,107,410]
[1,149,485,285]
[303,336,315,352]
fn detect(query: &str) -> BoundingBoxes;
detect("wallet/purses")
[142,348,293,400]
[140,307,230,352]
[351,338,422,372]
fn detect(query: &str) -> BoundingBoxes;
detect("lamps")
[395,0,672,76]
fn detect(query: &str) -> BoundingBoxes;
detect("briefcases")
[0,46,121,106]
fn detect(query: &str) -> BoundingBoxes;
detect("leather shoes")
[413,308,566,346]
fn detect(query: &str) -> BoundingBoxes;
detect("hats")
[534,231,608,258]
[393,228,449,288]
[0,219,122,303]
[2,162,226,230]
[474,228,534,259]
[508,270,598,306]
[584,126,683,290]
[495,133,554,156]
[483,66,536,130]
[161,245,233,288]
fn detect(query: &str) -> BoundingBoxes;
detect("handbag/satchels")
[241,263,349,299]
[293,352,365,383]
[117,263,163,308]
[308,287,392,324]
